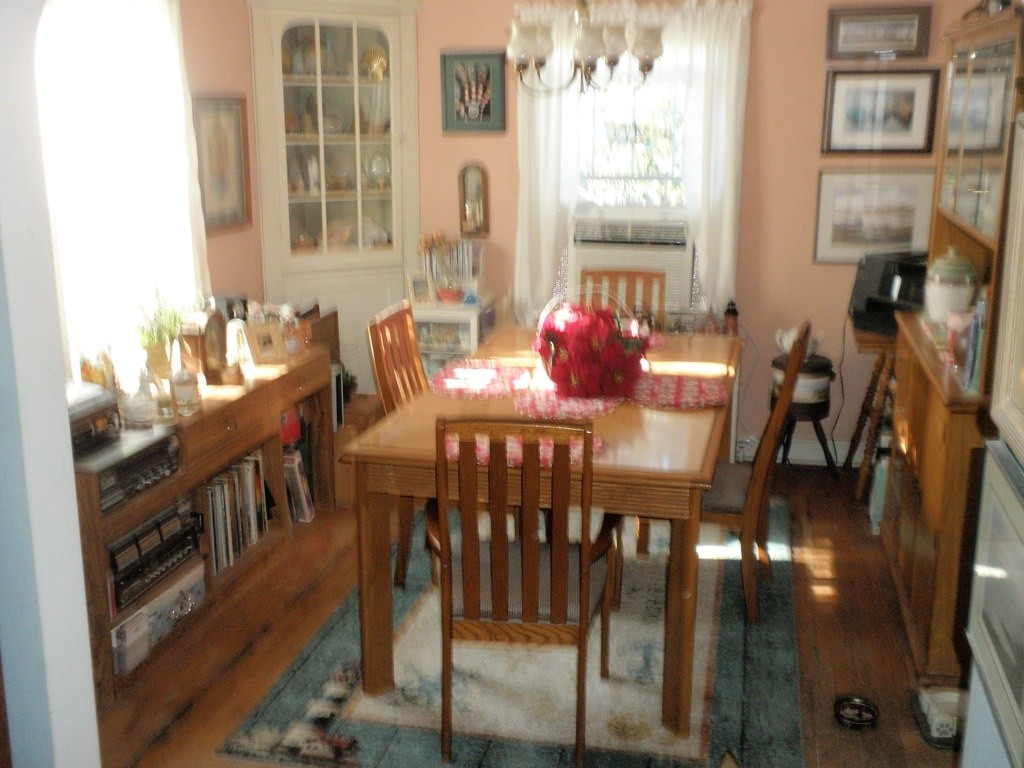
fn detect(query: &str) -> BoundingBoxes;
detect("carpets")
[215,495,802,768]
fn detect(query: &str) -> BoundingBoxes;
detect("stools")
[768,390,848,493]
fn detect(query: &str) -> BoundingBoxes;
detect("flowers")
[417,232,459,289]
[532,302,651,399]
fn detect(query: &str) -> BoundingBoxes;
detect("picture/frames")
[243,321,308,363]
[812,7,942,263]
[964,438,1024,768]
[406,271,437,308]
[194,97,252,238]
[440,51,507,136]
[941,67,1010,245]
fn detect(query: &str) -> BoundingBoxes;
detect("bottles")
[701,305,719,334]
[925,246,975,322]
[725,299,739,337]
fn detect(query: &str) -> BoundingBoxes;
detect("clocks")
[179,311,226,385]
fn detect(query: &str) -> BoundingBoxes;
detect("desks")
[340,324,746,741]
[341,391,384,501]
[844,316,896,507]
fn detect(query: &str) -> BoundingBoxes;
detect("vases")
[436,289,466,302]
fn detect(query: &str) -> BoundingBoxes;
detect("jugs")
[775,325,826,363]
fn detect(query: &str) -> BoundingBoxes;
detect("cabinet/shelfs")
[74,343,335,710]
[251,6,402,270]
[919,0,1024,399]
[879,313,999,685]
[407,296,495,380]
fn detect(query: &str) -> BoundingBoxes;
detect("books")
[419,239,477,281]
[193,450,270,576]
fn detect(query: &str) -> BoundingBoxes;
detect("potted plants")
[138,312,184,421]
[335,370,358,426]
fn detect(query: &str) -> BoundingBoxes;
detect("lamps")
[504,0,663,97]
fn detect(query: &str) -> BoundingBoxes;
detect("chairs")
[370,270,809,768]
